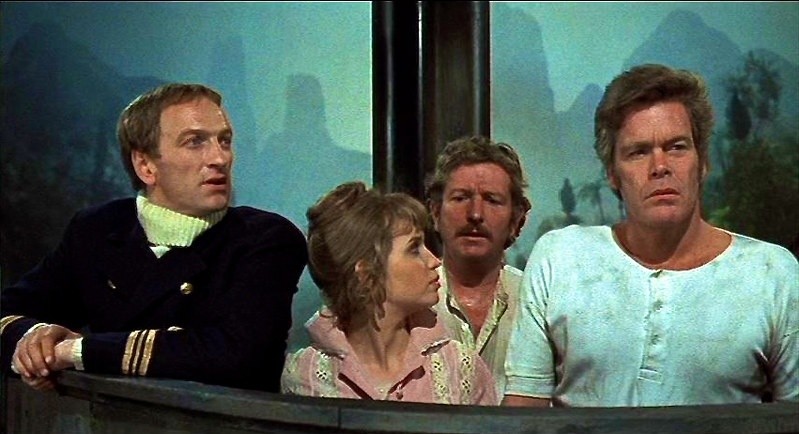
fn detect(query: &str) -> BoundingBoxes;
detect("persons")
[0,83,307,394]
[499,61,799,405]
[280,181,498,406]
[424,135,532,403]
